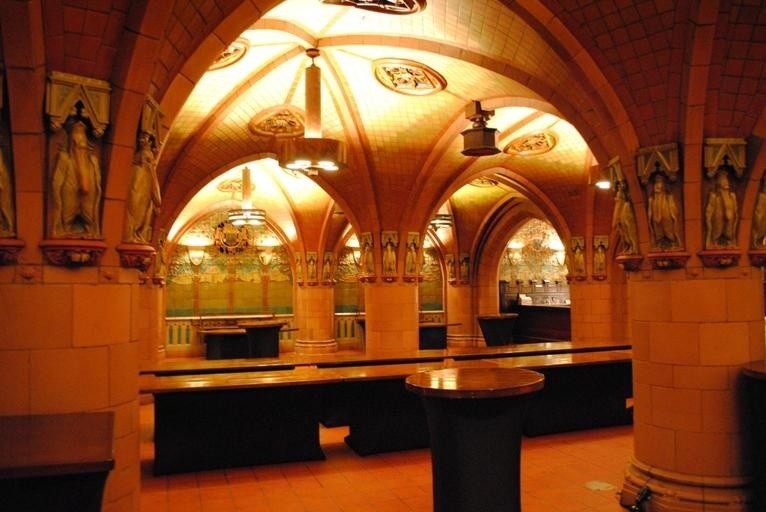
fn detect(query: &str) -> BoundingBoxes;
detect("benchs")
[0,410,117,512]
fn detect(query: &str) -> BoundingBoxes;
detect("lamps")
[227,168,266,227]
[429,213,453,232]
[277,49,348,177]
[460,116,501,157]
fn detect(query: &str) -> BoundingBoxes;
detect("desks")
[419,322,462,350]
[405,366,545,512]
[477,313,519,346]
[197,320,288,359]
